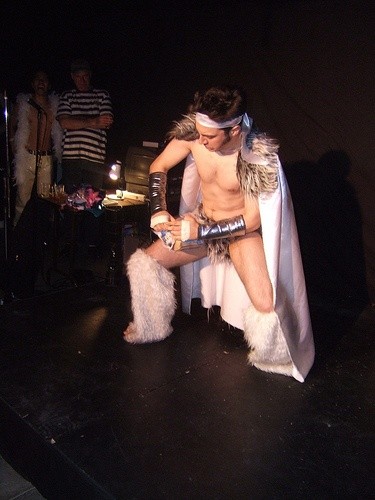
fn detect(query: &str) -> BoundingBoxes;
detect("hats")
[70,59,90,73]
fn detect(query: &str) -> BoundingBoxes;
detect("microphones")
[27,98,46,114]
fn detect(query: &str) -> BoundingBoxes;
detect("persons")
[123,90,293,348]
[8,67,61,228]
[53,55,113,189]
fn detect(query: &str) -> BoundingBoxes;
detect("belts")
[27,149,52,156]
